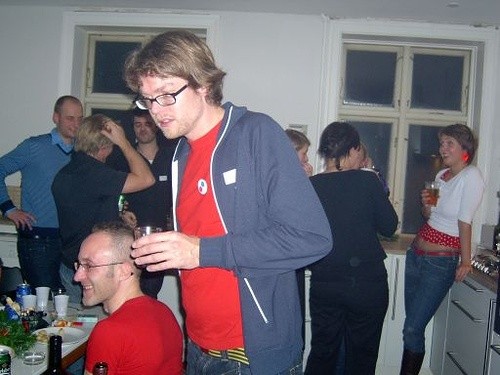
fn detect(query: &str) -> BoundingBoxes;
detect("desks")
[0,299,111,375]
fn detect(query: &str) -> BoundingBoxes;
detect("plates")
[32,327,86,346]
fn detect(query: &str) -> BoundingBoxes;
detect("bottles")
[95,361,108,375]
[41,335,64,375]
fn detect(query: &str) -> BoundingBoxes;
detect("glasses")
[73,258,124,273]
[133,81,190,109]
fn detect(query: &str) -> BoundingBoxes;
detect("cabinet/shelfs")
[302,251,500,375]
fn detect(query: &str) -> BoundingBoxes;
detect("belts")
[412,240,460,257]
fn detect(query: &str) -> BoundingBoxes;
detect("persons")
[123,31,332,375]
[74,222,183,375]
[400,125,487,375]
[0,96,84,294]
[304,122,398,375]
[284,130,313,178]
[117,107,176,296]
[52,114,155,375]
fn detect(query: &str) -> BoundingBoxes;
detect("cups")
[22,336,45,365]
[425,182,442,207]
[134,226,162,241]
[55,295,70,316]
[22,295,37,311]
[52,285,67,308]
[35,287,51,308]
